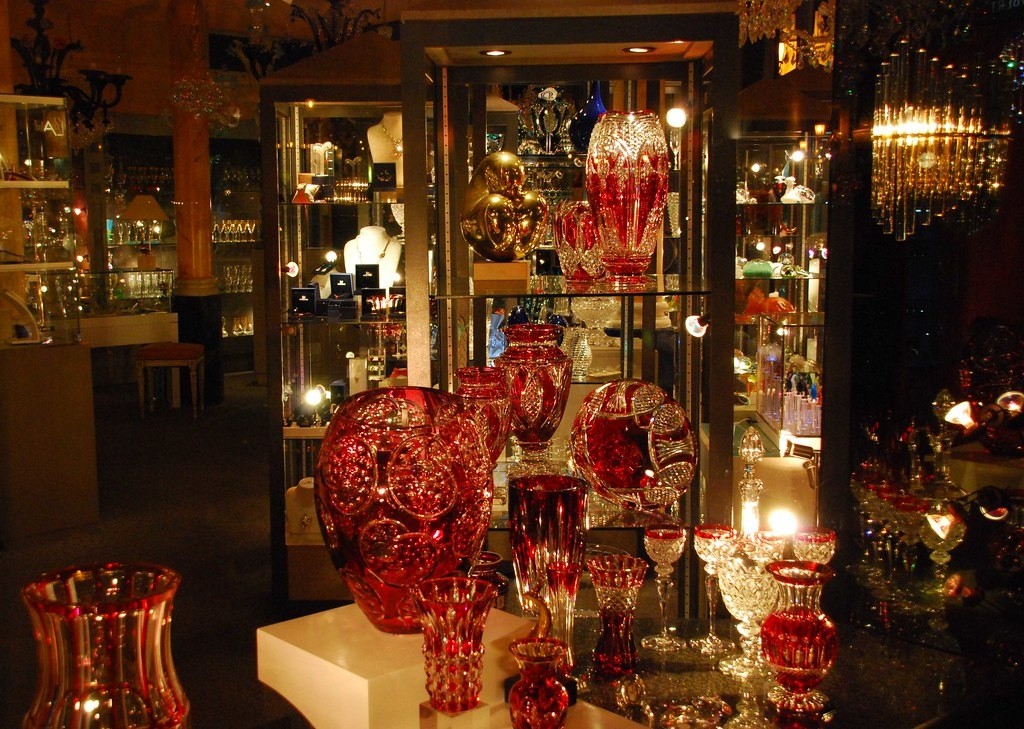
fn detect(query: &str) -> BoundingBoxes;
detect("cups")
[409,578,498,715]
[793,526,836,564]
[586,554,648,679]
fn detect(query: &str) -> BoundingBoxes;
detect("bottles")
[508,639,570,729]
[221,315,254,336]
[208,152,261,192]
[20,563,192,729]
[31,119,47,181]
[223,265,253,292]
[105,153,173,195]
[761,560,839,713]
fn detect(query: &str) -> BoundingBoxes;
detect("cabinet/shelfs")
[0,0,1024,729]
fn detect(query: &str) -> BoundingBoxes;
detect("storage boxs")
[373,162,397,191]
[328,300,358,320]
[760,310,825,437]
[312,175,333,202]
[353,264,380,294]
[308,282,328,316]
[328,274,354,300]
[472,259,532,297]
[289,287,317,321]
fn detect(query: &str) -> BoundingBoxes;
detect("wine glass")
[851,464,968,611]
[714,539,783,679]
[114,220,161,243]
[639,525,688,652]
[689,523,737,655]
[210,212,263,242]
[110,269,176,299]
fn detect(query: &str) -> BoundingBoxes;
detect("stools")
[134,342,207,421]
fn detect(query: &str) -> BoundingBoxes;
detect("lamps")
[864,20,1011,246]
[9,0,134,143]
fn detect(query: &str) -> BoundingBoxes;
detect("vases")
[19,559,195,729]
[585,76,672,286]
[589,554,649,679]
[552,188,606,282]
[507,474,592,670]
[507,636,569,729]
[500,322,575,454]
[454,363,515,471]
[315,386,495,631]
[759,558,840,716]
